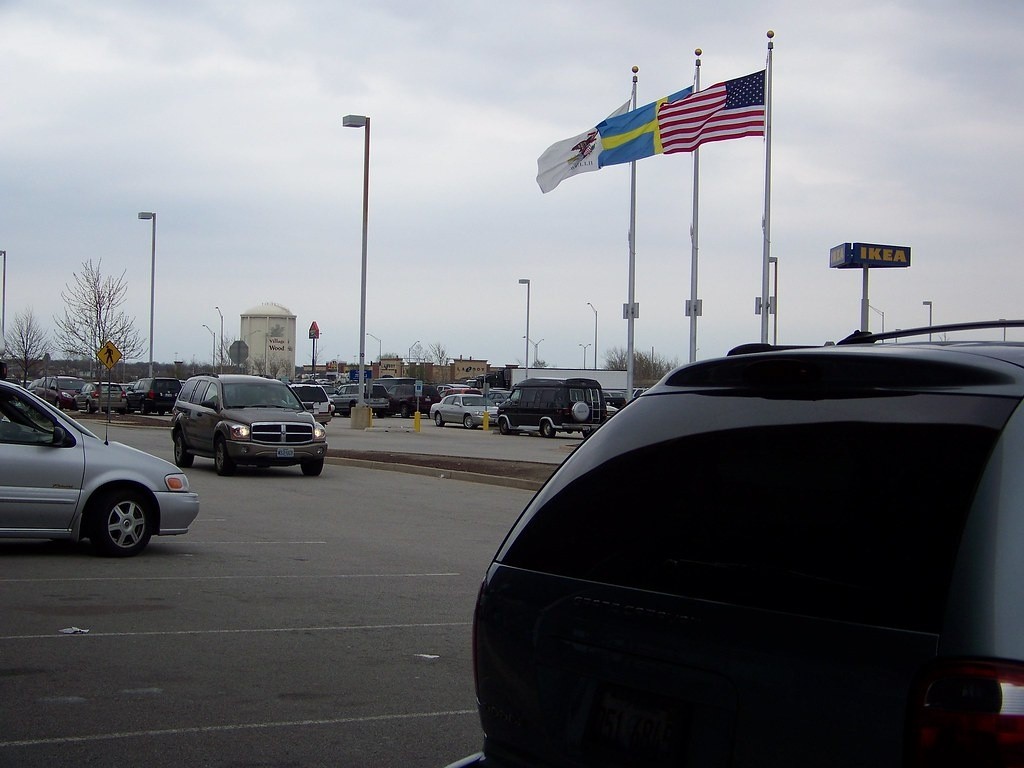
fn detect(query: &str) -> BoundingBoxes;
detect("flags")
[536,98,631,195]
[595,85,693,169]
[657,69,765,155]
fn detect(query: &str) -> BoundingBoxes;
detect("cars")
[599,387,649,418]
[73,381,128,416]
[0,380,201,559]
[434,373,521,409]
[0,377,34,406]
[294,371,358,400]
[430,393,499,430]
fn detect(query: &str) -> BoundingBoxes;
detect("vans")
[496,377,608,440]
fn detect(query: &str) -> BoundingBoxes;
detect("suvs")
[169,372,328,478]
[35,375,87,412]
[328,383,393,419]
[376,384,443,419]
[125,376,182,417]
[444,322,1023,768]
[281,380,336,428]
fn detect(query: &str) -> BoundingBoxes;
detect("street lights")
[579,344,592,370]
[0,250,7,340]
[203,324,215,374]
[215,306,224,374]
[408,340,421,365]
[366,333,381,359]
[342,115,372,410]
[588,303,598,370]
[523,335,545,361]
[768,255,780,346]
[518,279,531,379]
[137,211,157,377]
[922,301,932,342]
[998,319,1006,342]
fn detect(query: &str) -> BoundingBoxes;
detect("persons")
[261,389,286,403]
